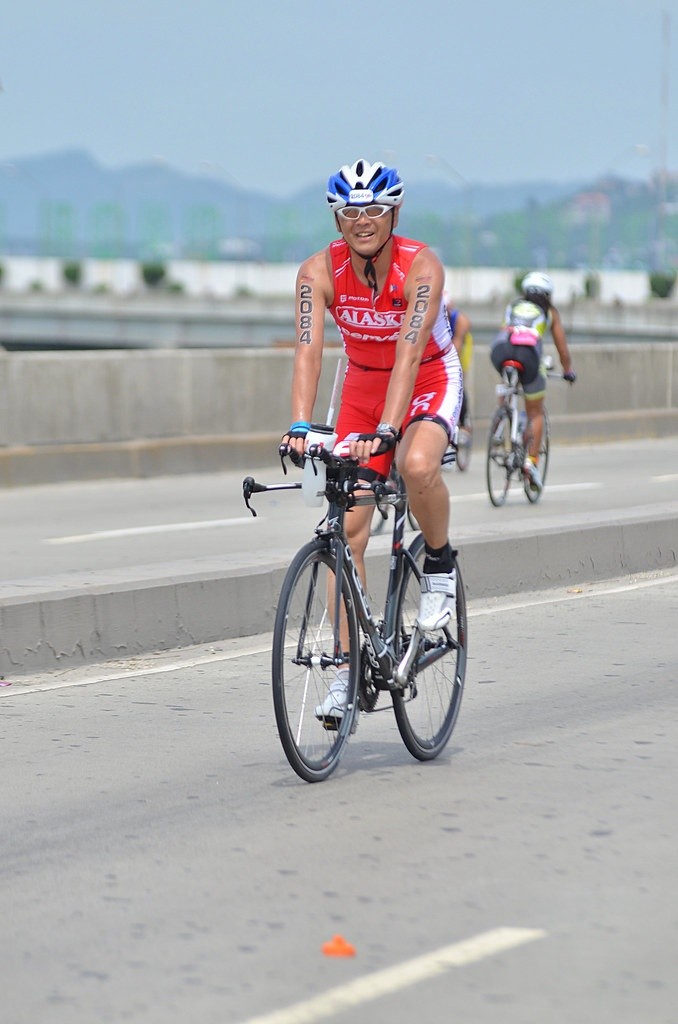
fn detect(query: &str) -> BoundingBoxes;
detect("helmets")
[326,158,406,211]
[521,271,553,293]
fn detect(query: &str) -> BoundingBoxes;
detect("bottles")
[301,423,337,507]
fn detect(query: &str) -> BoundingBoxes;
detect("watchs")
[377,423,399,437]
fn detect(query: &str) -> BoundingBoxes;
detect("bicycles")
[270,443,469,784]
[485,359,574,506]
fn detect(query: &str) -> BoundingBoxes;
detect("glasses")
[336,205,393,220]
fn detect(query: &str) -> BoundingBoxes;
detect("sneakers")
[521,455,543,494]
[314,679,358,734]
[414,568,457,631]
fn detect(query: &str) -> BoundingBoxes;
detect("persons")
[281,158,464,735]
[490,270,577,491]
[443,288,472,443]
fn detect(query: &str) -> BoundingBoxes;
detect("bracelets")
[290,421,311,430]
